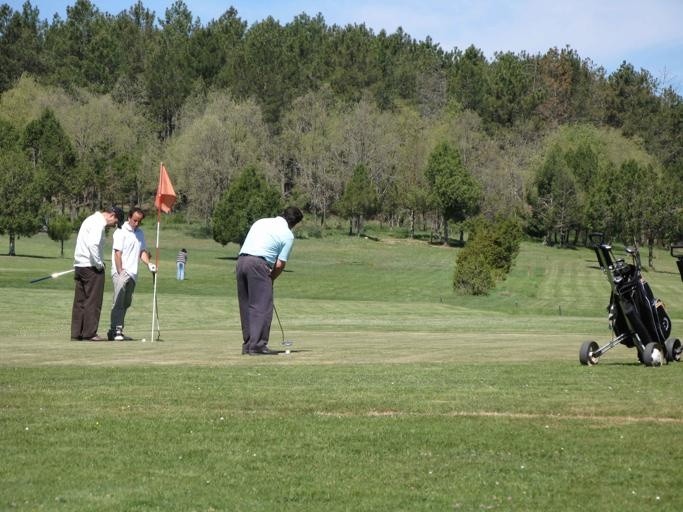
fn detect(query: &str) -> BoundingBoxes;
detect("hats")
[112,207,124,228]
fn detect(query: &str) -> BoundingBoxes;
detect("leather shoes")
[71,326,132,341]
[242,348,278,354]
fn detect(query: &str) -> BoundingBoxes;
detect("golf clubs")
[600,244,643,284]
[152,266,160,341]
[272,305,293,346]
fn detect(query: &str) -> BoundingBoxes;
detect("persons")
[108,208,160,342]
[233,205,304,355]
[68,205,125,344]
[175,247,188,281]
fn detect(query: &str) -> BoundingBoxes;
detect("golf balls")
[285,350,290,355]
[142,338,146,343]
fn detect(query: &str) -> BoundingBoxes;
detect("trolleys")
[579,232,683,367]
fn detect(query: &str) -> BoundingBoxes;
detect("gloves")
[148,263,157,272]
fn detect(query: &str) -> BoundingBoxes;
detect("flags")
[152,162,177,215]
